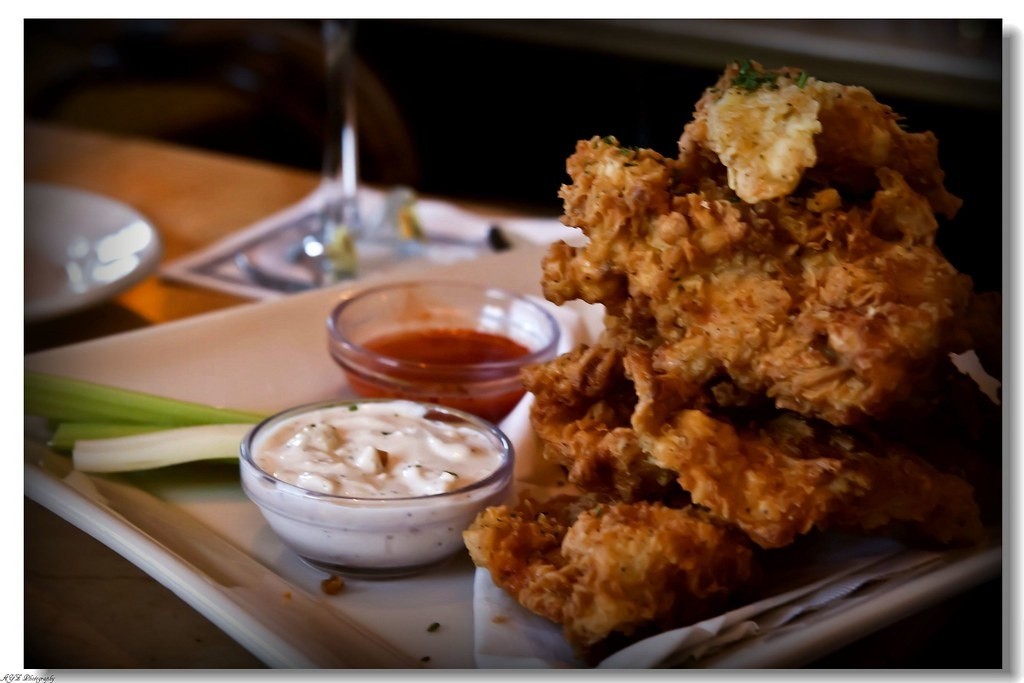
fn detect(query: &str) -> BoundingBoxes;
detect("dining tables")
[25,124,1004,671]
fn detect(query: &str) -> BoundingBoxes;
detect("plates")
[24,250,1002,669]
[24,184,159,319]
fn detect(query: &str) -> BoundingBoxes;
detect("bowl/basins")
[242,398,515,577]
[328,280,560,426]
